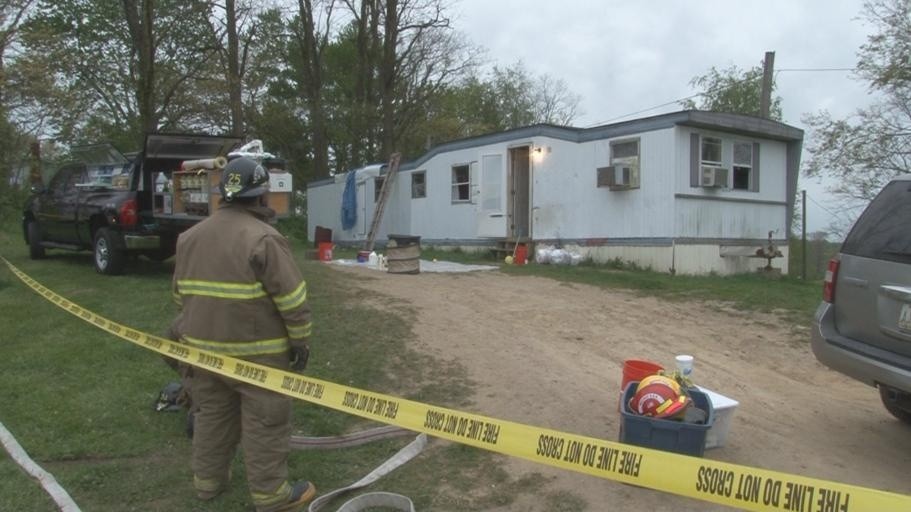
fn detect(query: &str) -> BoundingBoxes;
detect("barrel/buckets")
[385,234,421,275]
[318,242,333,260]
[155,172,168,193]
[618,359,664,411]
[357,250,369,263]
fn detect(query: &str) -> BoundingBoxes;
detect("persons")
[175,158,314,510]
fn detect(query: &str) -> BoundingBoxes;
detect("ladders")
[364,152,401,251]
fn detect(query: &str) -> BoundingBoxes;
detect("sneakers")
[257,481,315,512]
[198,469,233,500]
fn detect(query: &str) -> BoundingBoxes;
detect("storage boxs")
[695,384,738,448]
[619,380,713,457]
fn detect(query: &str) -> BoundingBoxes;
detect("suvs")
[810,171,911,423]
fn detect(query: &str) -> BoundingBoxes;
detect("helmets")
[628,374,692,419]
[220,157,270,201]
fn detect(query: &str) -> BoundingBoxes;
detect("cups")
[378,256,384,265]
[676,355,694,380]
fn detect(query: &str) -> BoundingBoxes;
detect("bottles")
[155,173,209,215]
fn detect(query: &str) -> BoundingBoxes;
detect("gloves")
[291,344,310,370]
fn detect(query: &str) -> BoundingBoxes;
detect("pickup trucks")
[19,125,253,276]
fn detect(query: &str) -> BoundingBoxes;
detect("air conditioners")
[702,165,728,187]
[596,165,632,188]
